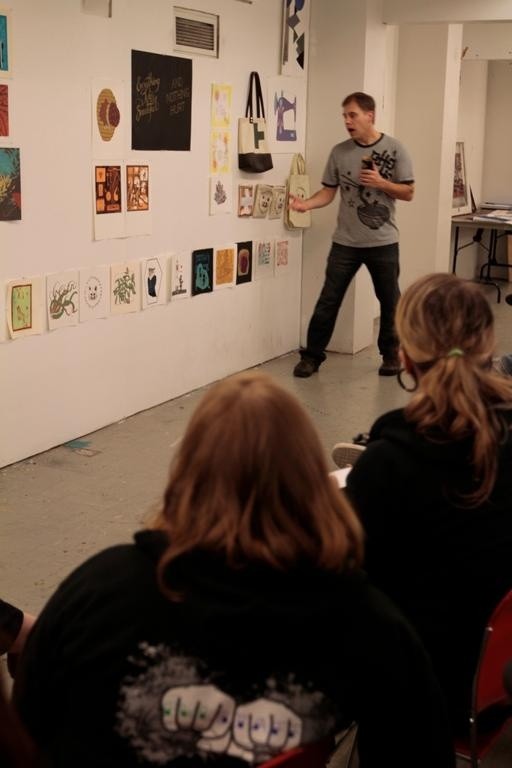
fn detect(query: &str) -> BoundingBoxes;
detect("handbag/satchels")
[238,72,274,174]
[284,152,312,230]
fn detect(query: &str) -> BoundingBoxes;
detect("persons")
[0,598,37,679]
[288,91,415,377]
[14,372,456,768]
[346,272,512,739]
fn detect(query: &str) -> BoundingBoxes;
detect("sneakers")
[379,361,401,376]
[332,431,369,468]
[294,359,319,377]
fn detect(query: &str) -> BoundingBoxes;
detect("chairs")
[454,589,512,768]
[255,740,329,768]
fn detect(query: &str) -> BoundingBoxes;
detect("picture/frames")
[451,137,472,217]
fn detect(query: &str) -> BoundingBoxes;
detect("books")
[473,214,511,224]
[480,203,511,209]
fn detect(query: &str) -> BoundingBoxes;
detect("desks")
[452,208,512,303]
